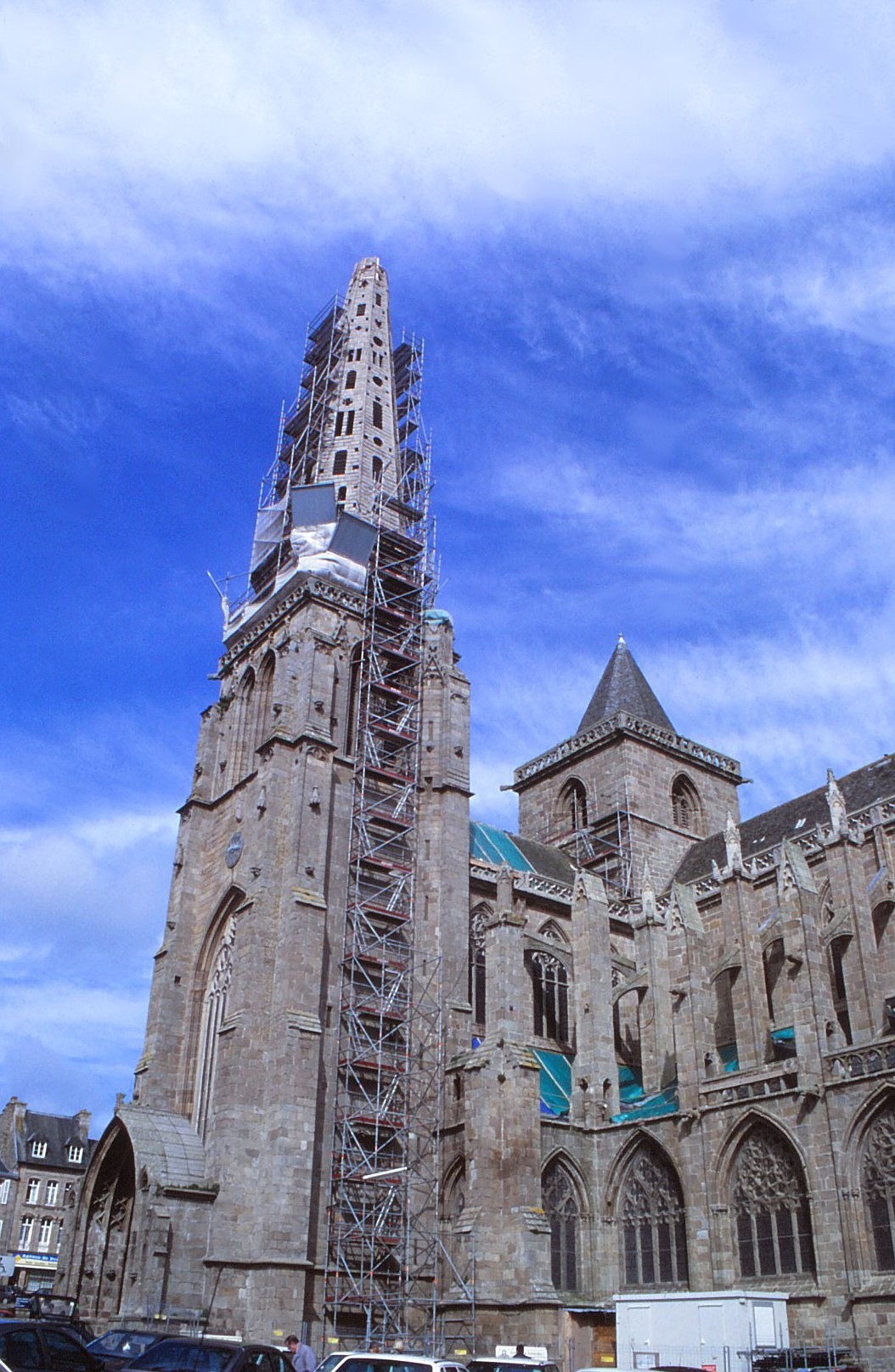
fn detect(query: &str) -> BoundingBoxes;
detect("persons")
[390,1339,404,1355]
[511,1345,531,1359]
[284,1334,315,1372]
[367,1341,379,1354]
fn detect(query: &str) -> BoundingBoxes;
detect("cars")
[77,1328,188,1372]
[0,1284,107,1372]
[313,1351,713,1372]
[120,1337,297,1372]
